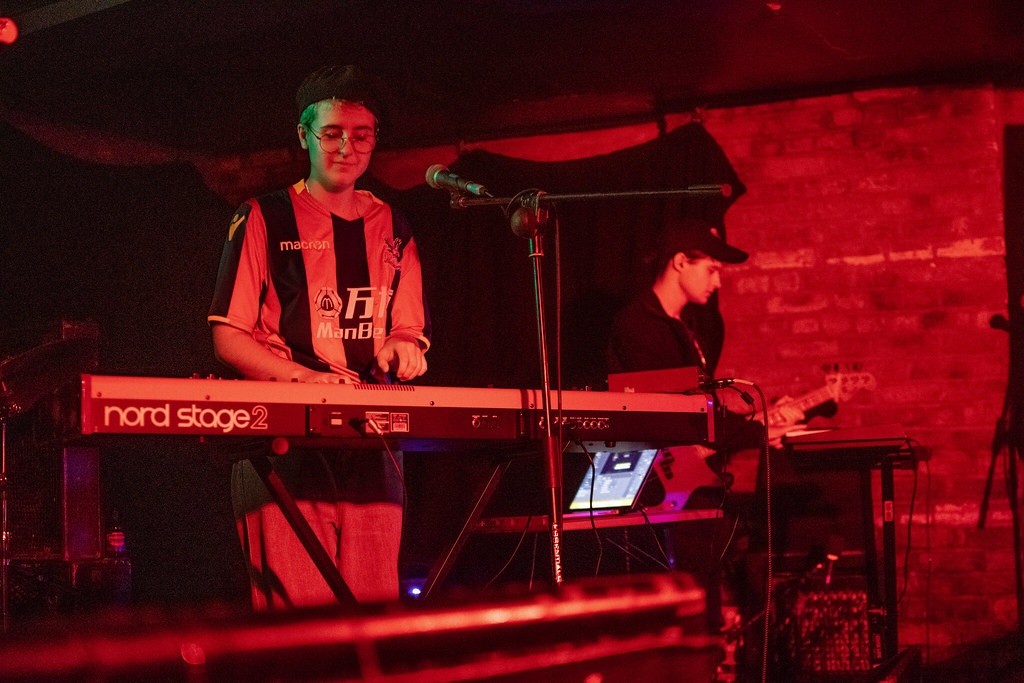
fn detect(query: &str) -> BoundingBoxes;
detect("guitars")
[597,360,876,515]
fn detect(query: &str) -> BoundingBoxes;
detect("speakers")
[0,401,104,564]
[787,576,884,674]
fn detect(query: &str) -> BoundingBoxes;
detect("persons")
[601,216,807,570]
[206,60,432,615]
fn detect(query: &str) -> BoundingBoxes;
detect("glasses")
[304,122,377,154]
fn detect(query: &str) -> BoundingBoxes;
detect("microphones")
[426,164,487,195]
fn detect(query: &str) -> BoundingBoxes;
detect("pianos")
[781,424,932,463]
[68,370,724,449]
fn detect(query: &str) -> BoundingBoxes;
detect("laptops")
[490,449,659,520]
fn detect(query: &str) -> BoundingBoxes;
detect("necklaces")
[654,283,707,368]
[304,179,361,221]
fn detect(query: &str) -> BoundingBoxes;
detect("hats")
[651,221,747,264]
[296,64,391,120]
